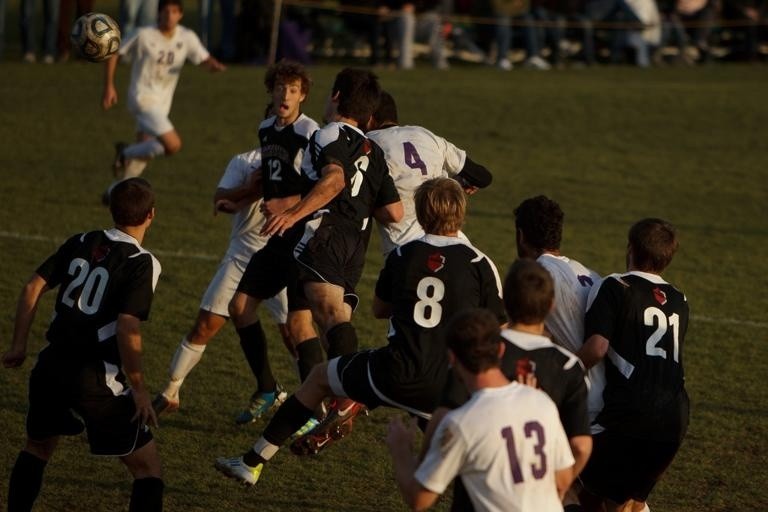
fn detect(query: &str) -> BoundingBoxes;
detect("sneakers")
[212,452,265,487]
[316,398,372,425]
[110,137,128,181]
[235,375,285,424]
[146,395,181,428]
[289,418,354,457]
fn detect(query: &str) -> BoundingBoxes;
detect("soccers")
[69,13,121,62]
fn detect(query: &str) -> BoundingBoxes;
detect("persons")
[99,0,226,207]
[216,0,768,72]
[150,63,689,511]
[18,0,158,66]
[5,179,167,512]
